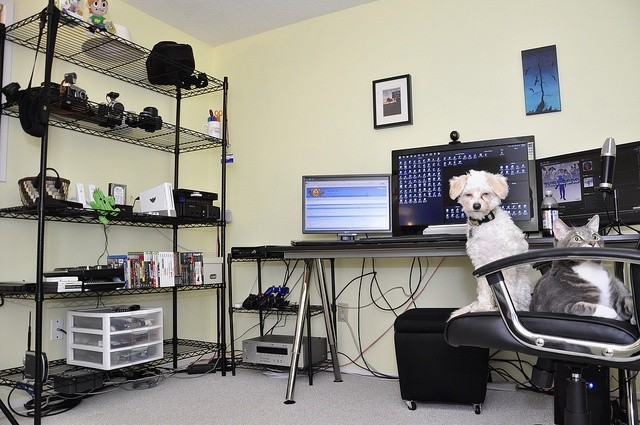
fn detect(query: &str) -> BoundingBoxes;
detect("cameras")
[125,106,163,133]
[95,91,126,130]
[40,72,88,114]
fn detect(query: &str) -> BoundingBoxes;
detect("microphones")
[598,136,618,187]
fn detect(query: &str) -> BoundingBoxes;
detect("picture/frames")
[522,44,561,116]
[374,74,412,129]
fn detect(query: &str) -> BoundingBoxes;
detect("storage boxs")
[67,304,163,371]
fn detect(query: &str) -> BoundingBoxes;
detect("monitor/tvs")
[536,141,640,233]
[300,173,392,240]
[391,135,538,235]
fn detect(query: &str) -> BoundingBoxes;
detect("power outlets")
[338,303,348,321]
[51,319,63,340]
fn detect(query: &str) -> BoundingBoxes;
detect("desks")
[285,235,619,400]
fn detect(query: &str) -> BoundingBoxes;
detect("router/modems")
[24,311,49,384]
[138,182,177,217]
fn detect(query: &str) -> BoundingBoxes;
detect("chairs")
[447,248,640,425]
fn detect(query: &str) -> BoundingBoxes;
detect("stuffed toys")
[61,0,83,26]
[89,189,122,224]
[87,0,116,38]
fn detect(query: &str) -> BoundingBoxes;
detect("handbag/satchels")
[18,168,70,206]
[18,6,60,137]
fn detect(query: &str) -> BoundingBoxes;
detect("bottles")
[541,191,559,237]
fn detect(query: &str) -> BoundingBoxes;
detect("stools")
[395,307,490,414]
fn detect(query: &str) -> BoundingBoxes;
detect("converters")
[188,364,216,375]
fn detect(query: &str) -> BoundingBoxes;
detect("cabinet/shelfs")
[222,257,337,386]
[0,0,228,425]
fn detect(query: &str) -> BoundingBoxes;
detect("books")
[107,251,203,288]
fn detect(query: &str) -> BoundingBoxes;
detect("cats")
[530,214,637,326]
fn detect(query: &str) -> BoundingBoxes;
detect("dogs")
[446,170,542,323]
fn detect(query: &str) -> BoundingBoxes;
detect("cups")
[202,120,221,139]
